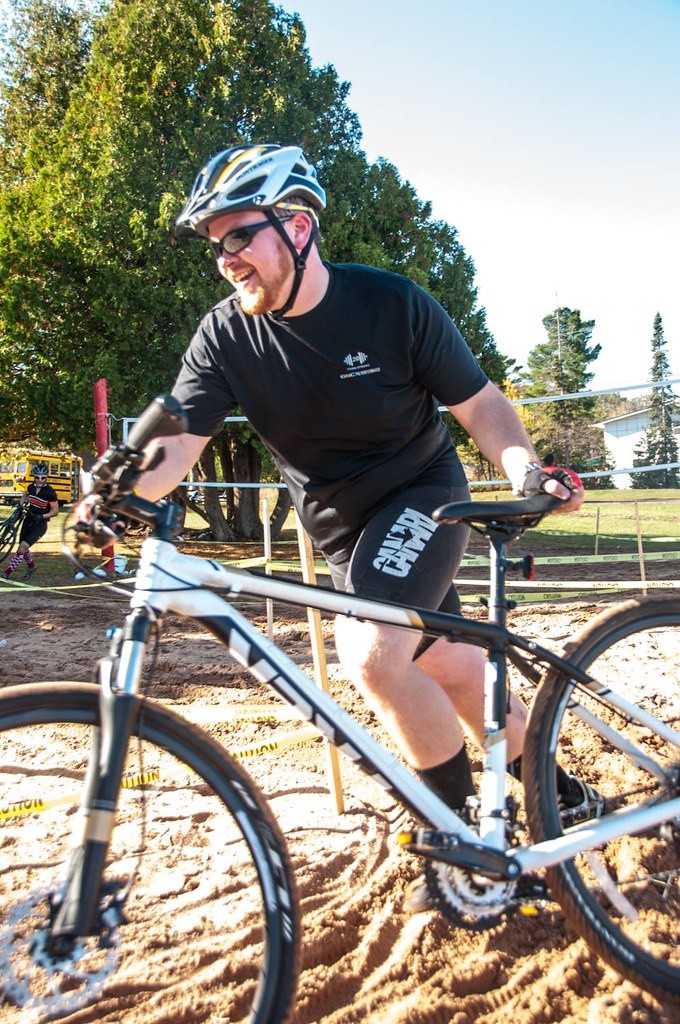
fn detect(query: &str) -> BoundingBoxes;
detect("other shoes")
[22,565,37,581]
[0,572,9,581]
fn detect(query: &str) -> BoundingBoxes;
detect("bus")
[0,448,84,508]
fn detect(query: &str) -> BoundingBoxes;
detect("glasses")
[33,476,47,480]
[210,215,298,260]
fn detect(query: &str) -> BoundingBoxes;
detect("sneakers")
[404,865,461,915]
[557,773,605,829]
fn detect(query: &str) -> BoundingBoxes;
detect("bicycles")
[0,392,679,1024]
[0,500,37,565]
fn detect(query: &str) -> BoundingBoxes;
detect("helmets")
[176,144,326,241]
[30,463,49,475]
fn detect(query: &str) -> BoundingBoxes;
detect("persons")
[0,463,59,581]
[76,145,606,911]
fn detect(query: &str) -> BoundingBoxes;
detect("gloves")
[33,514,43,524]
